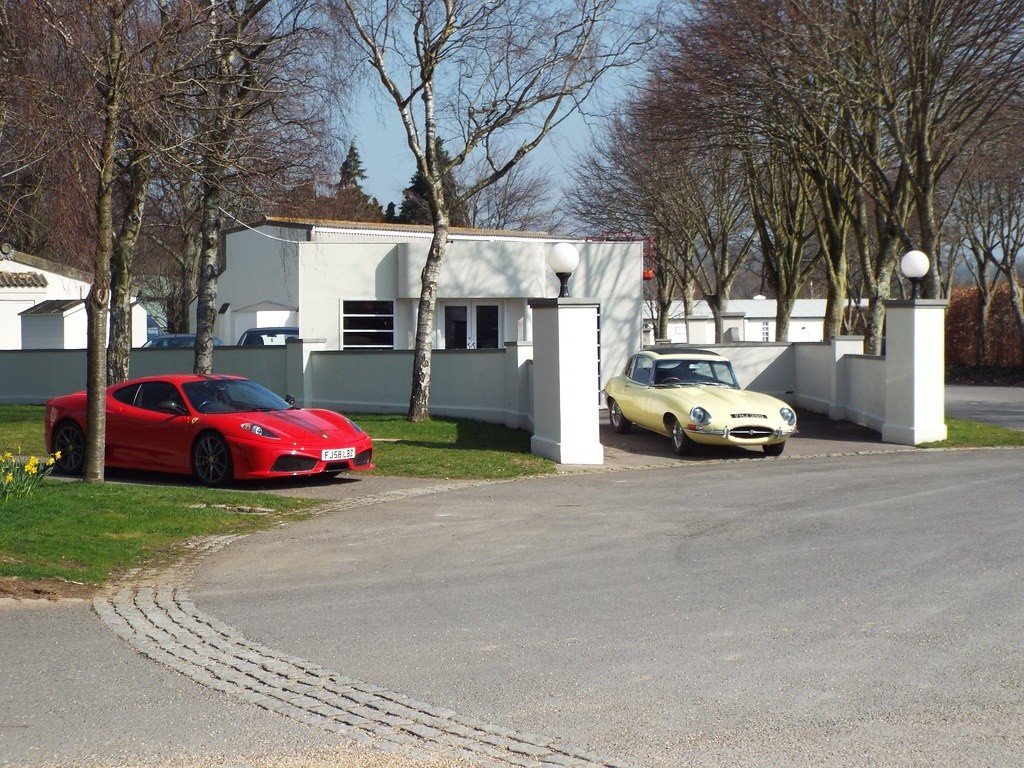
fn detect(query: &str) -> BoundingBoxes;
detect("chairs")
[628,367,699,385]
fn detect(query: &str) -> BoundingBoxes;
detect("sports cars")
[44,375,375,487]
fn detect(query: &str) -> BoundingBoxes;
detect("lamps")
[548,242,579,297]
[901,250,930,300]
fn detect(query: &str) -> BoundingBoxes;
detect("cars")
[603,349,797,456]
[141,317,224,348]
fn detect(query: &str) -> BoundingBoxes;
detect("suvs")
[237,327,299,347]
